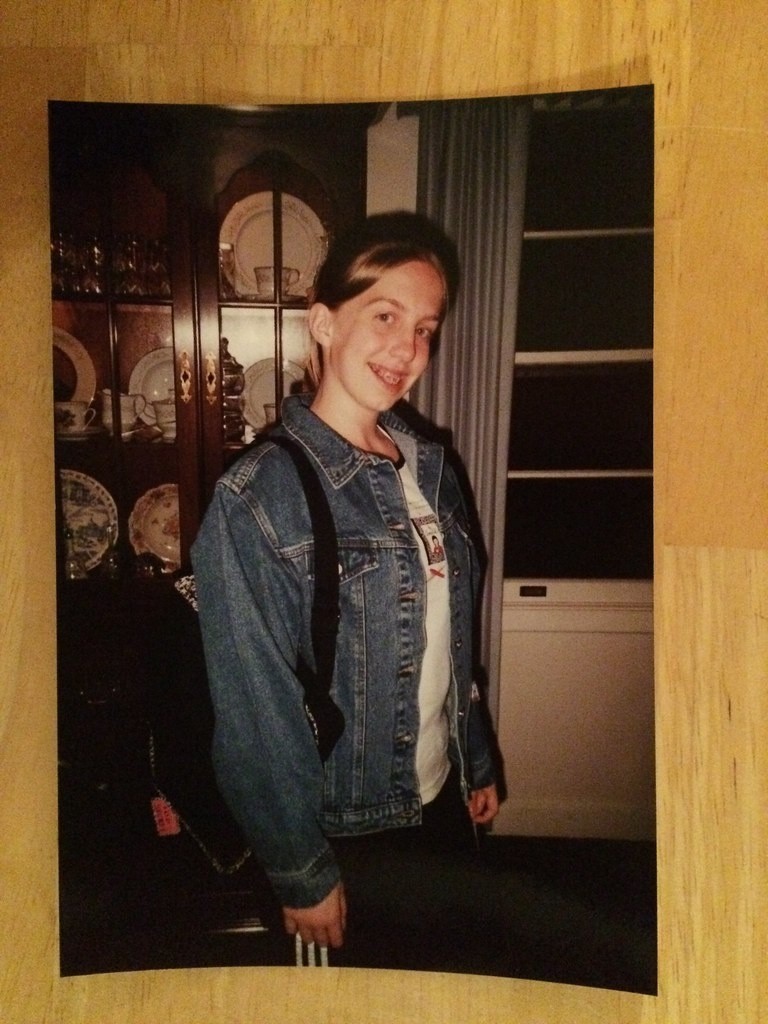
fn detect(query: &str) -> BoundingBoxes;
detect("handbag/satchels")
[105,428,346,870]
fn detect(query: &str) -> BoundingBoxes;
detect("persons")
[186,208,509,975]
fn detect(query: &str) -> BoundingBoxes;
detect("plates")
[221,189,331,297]
[127,346,176,432]
[58,468,118,573]
[53,326,96,409]
[240,357,306,429]
[126,483,181,574]
[56,433,94,444]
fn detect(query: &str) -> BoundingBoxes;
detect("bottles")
[63,530,88,586]
[102,526,122,578]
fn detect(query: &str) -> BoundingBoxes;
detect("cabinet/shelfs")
[48,102,368,937]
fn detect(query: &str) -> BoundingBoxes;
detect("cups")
[54,402,96,433]
[141,389,177,440]
[250,267,301,303]
[263,404,277,425]
[52,222,173,303]
[99,388,145,436]
